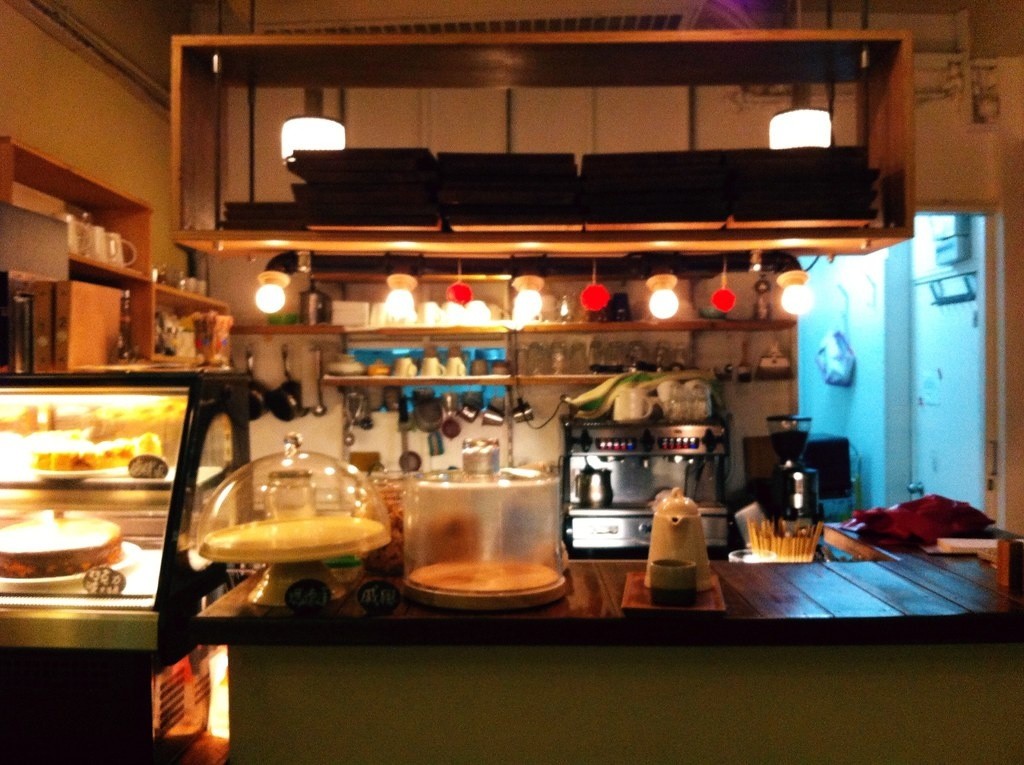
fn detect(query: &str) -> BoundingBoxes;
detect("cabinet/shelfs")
[168,0,915,257]
[2,373,252,612]
[225,257,799,383]
[0,134,233,374]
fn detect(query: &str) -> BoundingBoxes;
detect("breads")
[28,430,161,471]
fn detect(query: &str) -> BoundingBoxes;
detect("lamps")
[767,81,832,150]
[278,87,347,164]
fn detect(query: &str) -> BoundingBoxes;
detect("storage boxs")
[34,277,125,371]
[741,431,851,498]
[1,176,74,281]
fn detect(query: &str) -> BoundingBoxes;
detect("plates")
[0,540,143,586]
[27,469,129,481]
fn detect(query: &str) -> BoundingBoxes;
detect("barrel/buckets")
[299,290,331,326]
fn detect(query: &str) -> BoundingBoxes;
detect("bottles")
[108,289,138,365]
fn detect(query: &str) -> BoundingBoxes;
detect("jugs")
[445,357,468,376]
[62,215,136,273]
[393,357,418,376]
[612,390,653,424]
[575,469,613,509]
[421,358,446,376]
[641,487,713,591]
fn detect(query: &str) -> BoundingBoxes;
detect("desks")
[193,557,1023,763]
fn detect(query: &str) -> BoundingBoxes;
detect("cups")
[510,398,534,424]
[482,406,504,427]
[516,339,691,375]
[649,559,697,608]
[727,549,778,562]
[1010,541,1024,597]
[455,403,479,424]
[470,360,488,376]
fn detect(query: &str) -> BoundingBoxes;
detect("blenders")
[765,415,821,520]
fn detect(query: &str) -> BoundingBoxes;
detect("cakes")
[0,518,122,579]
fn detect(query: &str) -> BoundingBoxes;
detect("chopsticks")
[745,517,824,562]
